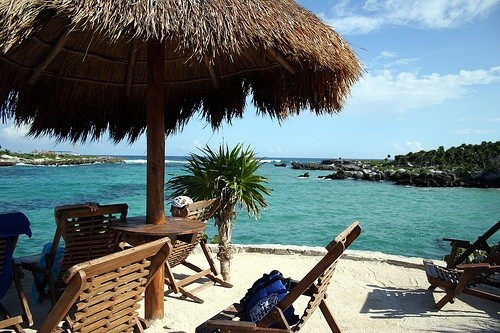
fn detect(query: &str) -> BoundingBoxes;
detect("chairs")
[157,196,233,303]
[36,236,174,333]
[17,203,129,313]
[0,211,34,333]
[195,222,362,333]
[423,220,500,310]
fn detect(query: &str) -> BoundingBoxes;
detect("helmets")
[172,195,193,208]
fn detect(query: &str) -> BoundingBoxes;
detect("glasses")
[171,205,183,210]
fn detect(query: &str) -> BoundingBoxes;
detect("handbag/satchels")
[244,269,294,328]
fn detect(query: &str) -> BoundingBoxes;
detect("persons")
[168,195,193,218]
[42,200,105,283]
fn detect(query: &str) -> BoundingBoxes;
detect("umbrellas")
[0,0,368,320]
